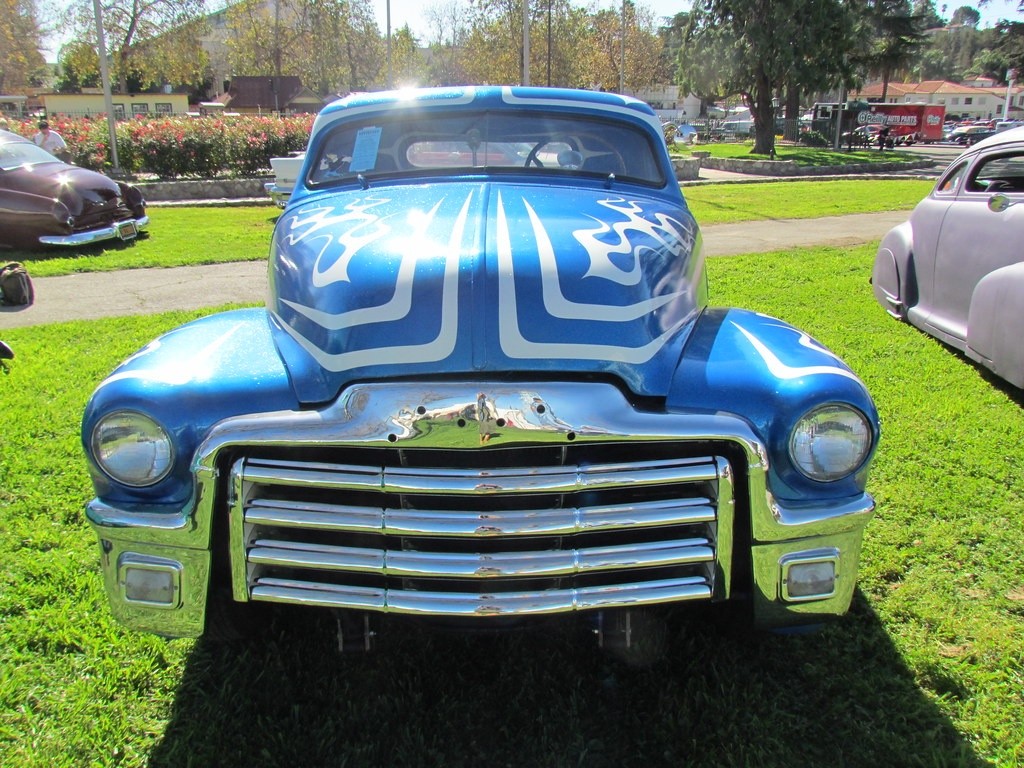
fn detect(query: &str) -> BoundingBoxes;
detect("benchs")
[725,137,736,143]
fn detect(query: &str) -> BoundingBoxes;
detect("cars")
[0,129,149,253]
[870,125,1024,394]
[842,124,897,146]
[942,118,1024,146]
[79,82,880,660]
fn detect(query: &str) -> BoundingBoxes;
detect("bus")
[809,102,946,146]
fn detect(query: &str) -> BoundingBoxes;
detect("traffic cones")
[966,138,970,148]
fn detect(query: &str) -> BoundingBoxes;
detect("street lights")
[771,97,780,133]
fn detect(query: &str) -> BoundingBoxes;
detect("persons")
[477,393,491,442]
[0,118,8,131]
[863,123,872,149]
[34,121,67,156]
[878,128,889,151]
[989,120,995,129]
